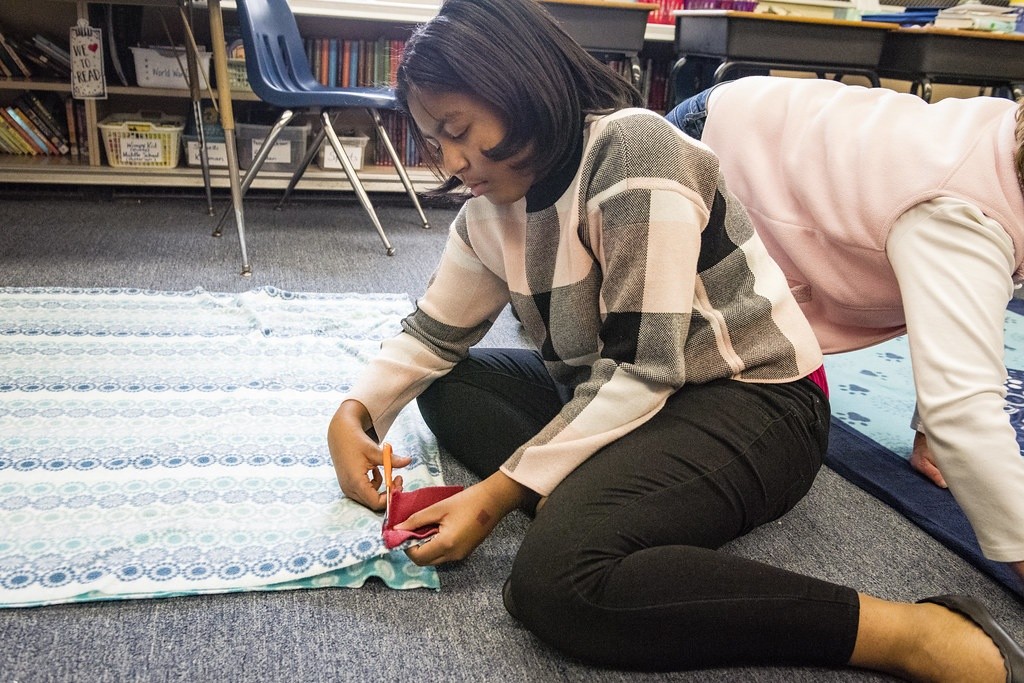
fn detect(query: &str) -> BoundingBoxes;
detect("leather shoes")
[914,594,1022,683]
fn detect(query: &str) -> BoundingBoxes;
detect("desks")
[665,9,900,119]
[876,26,1024,104]
[535,0,660,63]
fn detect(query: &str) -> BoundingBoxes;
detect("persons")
[327,0,1024,683]
[664,75,1022,578]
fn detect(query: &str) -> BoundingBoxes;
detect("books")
[1,28,632,168]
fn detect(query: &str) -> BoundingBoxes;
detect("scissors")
[382,441,392,518]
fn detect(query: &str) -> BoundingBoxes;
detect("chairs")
[212,0,431,258]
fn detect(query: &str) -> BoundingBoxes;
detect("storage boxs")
[311,129,370,172]
[234,111,312,173]
[226,58,253,93]
[128,45,214,90]
[96,109,187,170]
[180,120,229,171]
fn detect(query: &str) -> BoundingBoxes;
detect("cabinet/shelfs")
[0,0,472,196]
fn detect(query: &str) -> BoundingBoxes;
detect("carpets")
[0,286,448,611]
[820,294,1024,604]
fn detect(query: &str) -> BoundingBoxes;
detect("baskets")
[227,58,254,91]
[97,107,185,168]
